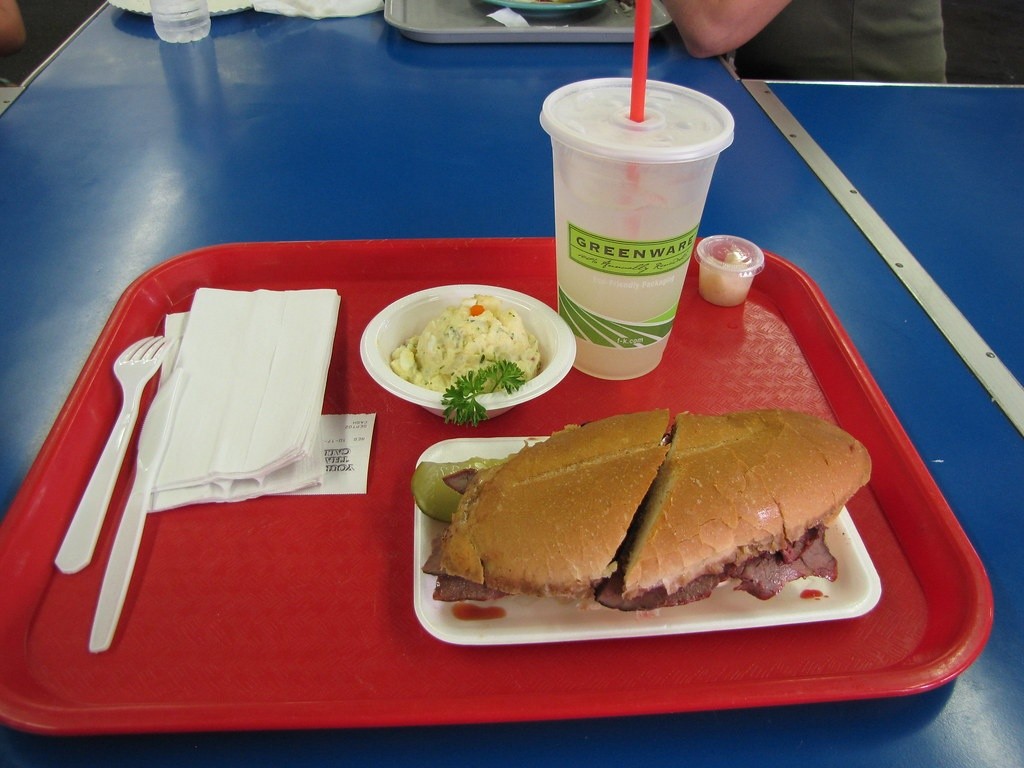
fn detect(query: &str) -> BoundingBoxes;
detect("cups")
[539,76,735,381]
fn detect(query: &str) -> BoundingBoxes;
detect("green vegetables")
[440,358,526,427]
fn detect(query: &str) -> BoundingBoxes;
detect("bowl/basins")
[359,282,578,422]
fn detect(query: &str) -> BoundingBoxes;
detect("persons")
[664,1,948,84]
[0,0,27,54]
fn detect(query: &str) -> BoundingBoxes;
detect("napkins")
[138,284,340,515]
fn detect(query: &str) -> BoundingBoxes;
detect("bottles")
[148,0,211,43]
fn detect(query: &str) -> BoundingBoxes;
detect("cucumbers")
[410,457,514,522]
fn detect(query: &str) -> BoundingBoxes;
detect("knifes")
[88,366,188,654]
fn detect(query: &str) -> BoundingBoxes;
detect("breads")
[593,410,871,594]
[439,407,672,590]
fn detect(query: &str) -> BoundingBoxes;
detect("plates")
[477,0,611,19]
[107,0,261,17]
[412,436,882,647]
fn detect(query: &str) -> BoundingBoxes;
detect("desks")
[0,0,1024,768]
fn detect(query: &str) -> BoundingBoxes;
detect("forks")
[54,335,179,574]
